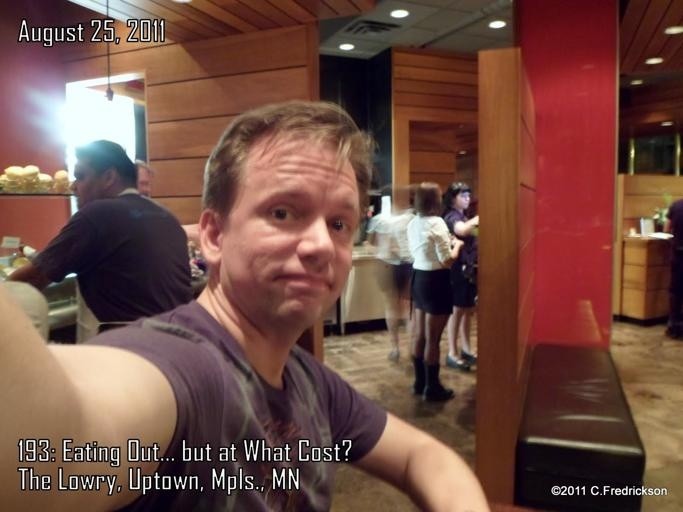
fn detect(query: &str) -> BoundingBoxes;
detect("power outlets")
[0,191,86,344]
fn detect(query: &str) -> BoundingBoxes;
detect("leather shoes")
[445,349,477,370]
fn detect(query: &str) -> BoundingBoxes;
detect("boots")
[410,354,455,401]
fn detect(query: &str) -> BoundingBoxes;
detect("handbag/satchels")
[452,245,475,278]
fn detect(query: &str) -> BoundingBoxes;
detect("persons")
[367,178,481,405]
[0,100,492,512]
[660,198,683,341]
[4,140,193,334]
[133,160,151,198]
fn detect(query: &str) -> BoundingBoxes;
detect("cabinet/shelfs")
[620,236,674,320]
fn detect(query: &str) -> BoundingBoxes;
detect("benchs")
[512,343,645,512]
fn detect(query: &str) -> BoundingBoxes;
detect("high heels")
[387,350,400,362]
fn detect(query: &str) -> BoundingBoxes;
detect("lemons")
[0,165,68,195]
